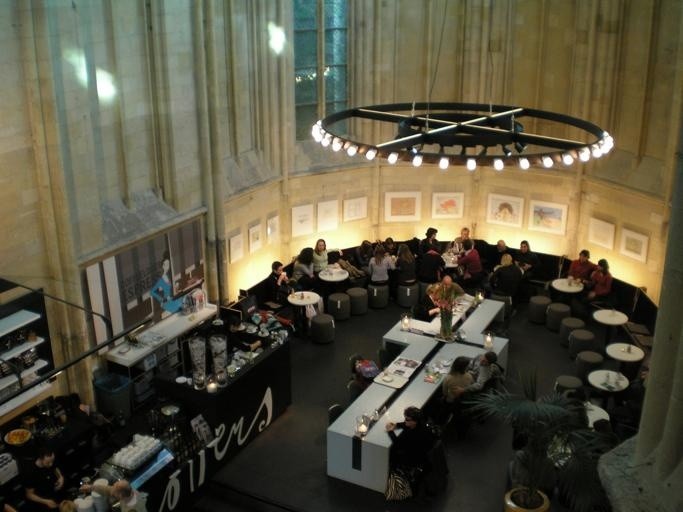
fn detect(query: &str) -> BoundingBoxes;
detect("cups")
[476,288,485,302]
[187,378,192,386]
[355,414,370,436]
[111,435,160,466]
[281,272,286,277]
[400,312,411,330]
[482,330,494,349]
[227,365,236,377]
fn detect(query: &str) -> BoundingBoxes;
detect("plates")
[73,478,109,511]
[4,429,32,446]
[231,325,269,338]
[175,376,186,385]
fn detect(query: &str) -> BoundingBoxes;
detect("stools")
[240,240,654,504]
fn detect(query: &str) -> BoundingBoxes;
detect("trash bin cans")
[93,373,131,421]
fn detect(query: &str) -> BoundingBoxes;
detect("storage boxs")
[130,336,180,406]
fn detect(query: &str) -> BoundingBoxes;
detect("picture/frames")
[383,190,423,224]
[429,190,465,220]
[228,195,370,268]
[617,225,650,264]
[484,192,525,230]
[527,198,570,236]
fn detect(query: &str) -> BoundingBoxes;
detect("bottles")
[425,363,429,372]
[233,352,241,369]
[300,292,303,299]
[290,288,295,298]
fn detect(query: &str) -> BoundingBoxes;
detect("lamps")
[307,0,618,174]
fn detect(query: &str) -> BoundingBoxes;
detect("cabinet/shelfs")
[0,289,54,415]
[102,300,219,414]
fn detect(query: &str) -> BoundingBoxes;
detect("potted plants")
[456,367,606,512]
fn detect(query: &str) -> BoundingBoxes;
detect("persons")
[584,259,612,305]
[566,389,611,450]
[613,360,651,408]
[440,356,474,412]
[450,352,504,399]
[569,250,598,297]
[269,228,540,322]
[387,408,431,477]
[0,446,146,512]
[151,258,183,313]
[203,315,261,373]
